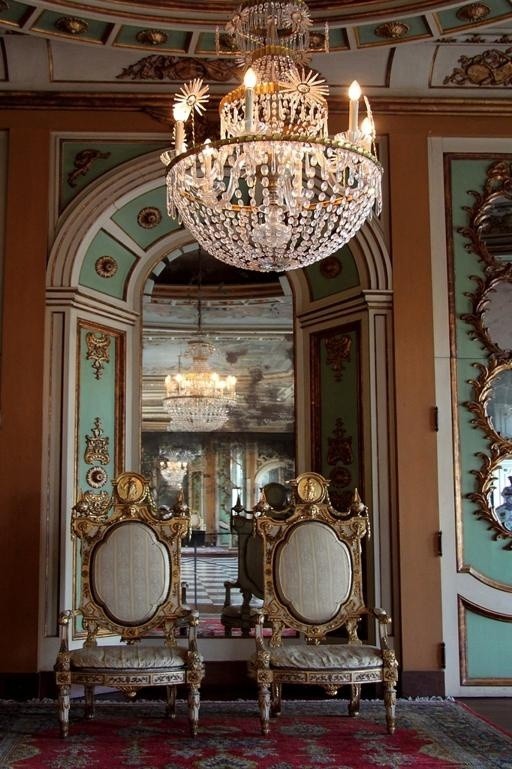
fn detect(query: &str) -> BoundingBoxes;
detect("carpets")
[1,697,511,769]
[144,618,298,638]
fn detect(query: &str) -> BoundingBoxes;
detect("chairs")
[242,474,399,739]
[221,482,289,638]
[51,473,208,742]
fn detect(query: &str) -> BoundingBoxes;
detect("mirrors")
[138,240,294,624]
[455,157,512,554]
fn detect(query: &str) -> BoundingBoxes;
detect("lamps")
[169,2,386,280]
[162,246,239,433]
